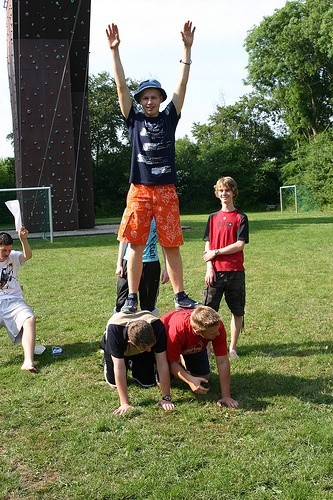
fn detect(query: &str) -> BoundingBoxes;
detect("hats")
[133,79,167,105]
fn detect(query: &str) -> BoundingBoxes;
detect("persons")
[106,20,199,314]
[114,219,169,312]
[0,226,37,371]
[203,177,249,355]
[99,310,174,416]
[156,305,238,407]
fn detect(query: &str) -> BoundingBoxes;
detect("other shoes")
[20,363,36,372]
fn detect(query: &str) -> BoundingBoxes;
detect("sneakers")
[121,293,138,314]
[173,291,202,310]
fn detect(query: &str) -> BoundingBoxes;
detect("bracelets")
[215,249,218,255]
[179,58,192,64]
